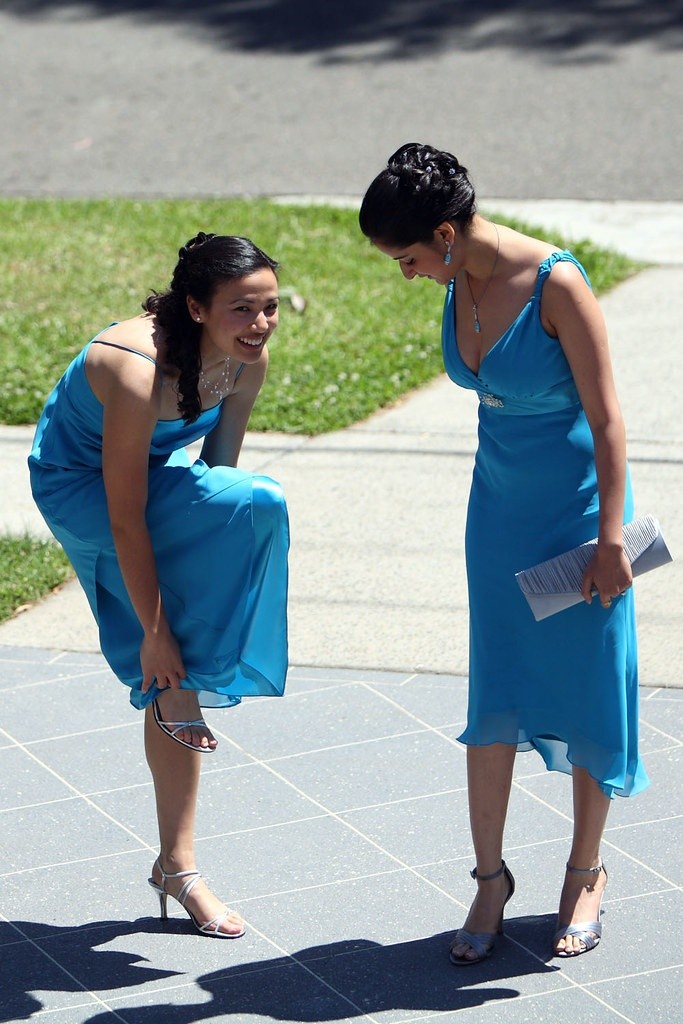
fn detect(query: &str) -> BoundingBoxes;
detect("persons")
[359,142,646,964]
[27,232,290,939]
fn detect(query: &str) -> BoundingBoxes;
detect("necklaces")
[467,223,499,333]
[200,358,229,400]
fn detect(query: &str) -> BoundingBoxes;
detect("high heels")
[153,692,217,754]
[557,858,608,957]
[450,859,515,965]
[148,859,247,939]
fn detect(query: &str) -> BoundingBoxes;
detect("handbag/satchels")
[514,514,673,622]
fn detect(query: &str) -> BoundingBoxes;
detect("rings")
[621,591,625,595]
[601,602,611,608]
